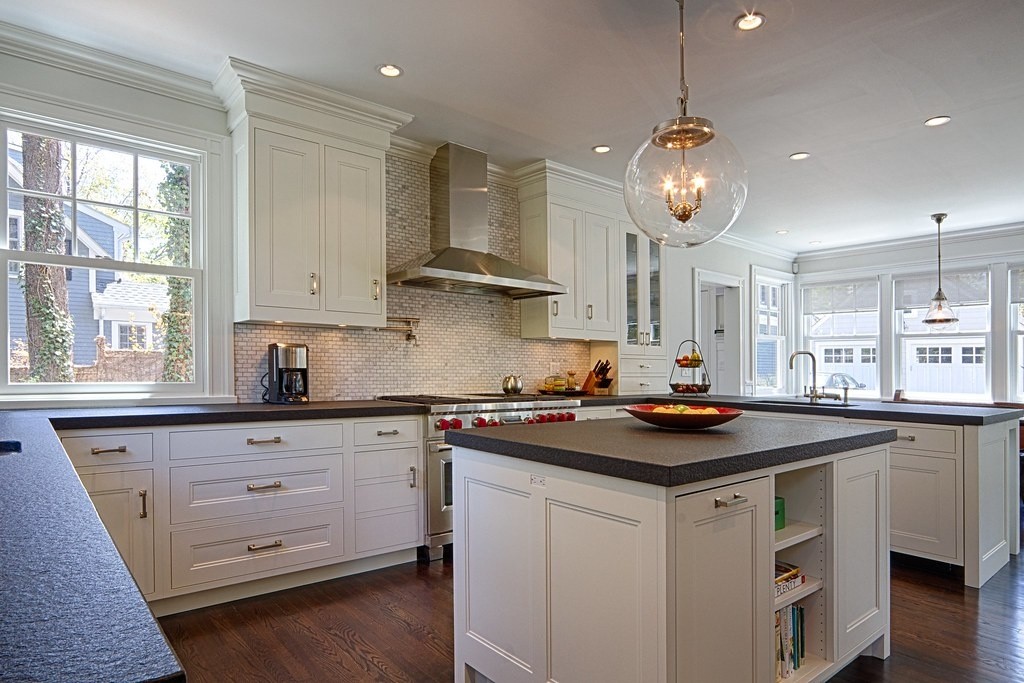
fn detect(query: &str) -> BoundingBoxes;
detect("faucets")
[789,352,817,402]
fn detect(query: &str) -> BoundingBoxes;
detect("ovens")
[423,438,453,549]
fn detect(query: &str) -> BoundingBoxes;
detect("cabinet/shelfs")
[836,445,890,665]
[58,426,159,602]
[546,193,584,340]
[619,221,670,395]
[323,135,387,327]
[161,419,348,599]
[585,209,619,339]
[246,114,321,324]
[848,421,966,567]
[772,456,836,682]
[662,469,769,683]
[349,416,426,561]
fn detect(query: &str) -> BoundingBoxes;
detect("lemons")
[653,404,721,414]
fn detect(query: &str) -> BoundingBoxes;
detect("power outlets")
[549,361,560,377]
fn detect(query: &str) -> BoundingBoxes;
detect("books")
[774,604,806,683]
[775,559,805,597]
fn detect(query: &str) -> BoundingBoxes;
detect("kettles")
[502,374,523,396]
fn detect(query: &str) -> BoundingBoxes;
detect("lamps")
[625,1,749,249]
[923,212,960,325]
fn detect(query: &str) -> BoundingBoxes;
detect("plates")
[622,404,743,430]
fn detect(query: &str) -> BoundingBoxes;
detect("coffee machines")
[260,343,310,405]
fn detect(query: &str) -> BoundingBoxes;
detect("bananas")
[689,349,701,368]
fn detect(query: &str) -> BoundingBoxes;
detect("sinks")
[750,400,856,407]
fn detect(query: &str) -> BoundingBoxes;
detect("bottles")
[566,371,576,392]
[553,379,566,392]
[545,377,554,391]
[575,382,581,391]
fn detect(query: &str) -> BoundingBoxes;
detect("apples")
[676,354,691,367]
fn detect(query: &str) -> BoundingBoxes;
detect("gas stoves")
[378,393,581,439]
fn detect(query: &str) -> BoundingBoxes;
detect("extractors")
[386,143,568,300]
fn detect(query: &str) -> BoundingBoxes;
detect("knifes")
[593,360,614,388]
